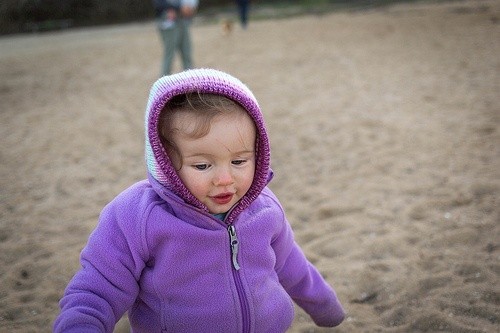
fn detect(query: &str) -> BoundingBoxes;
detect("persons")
[54,68,346,333]
[157,0,199,76]
